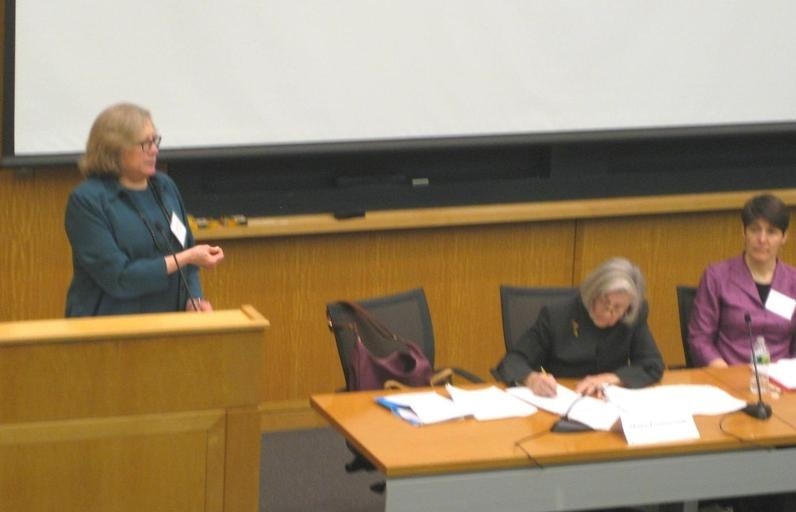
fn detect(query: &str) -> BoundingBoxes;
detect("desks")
[704,360,796,430]
[311,368,796,512]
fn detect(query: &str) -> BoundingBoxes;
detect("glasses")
[133,135,162,153]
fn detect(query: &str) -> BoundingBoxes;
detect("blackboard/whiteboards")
[157,121,796,241]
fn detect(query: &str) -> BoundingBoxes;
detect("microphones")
[745,313,771,419]
[553,387,588,432]
[154,219,197,311]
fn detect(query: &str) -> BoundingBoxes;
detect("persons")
[685,194,796,368]
[65,103,225,316]
[496,258,666,400]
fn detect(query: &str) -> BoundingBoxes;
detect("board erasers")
[334,208,367,220]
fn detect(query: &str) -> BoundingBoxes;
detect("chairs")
[327,288,484,494]
[499,284,580,355]
[677,286,709,367]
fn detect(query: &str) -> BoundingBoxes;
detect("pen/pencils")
[540,365,548,376]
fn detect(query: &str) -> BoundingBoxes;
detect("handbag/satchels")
[325,300,432,391]
